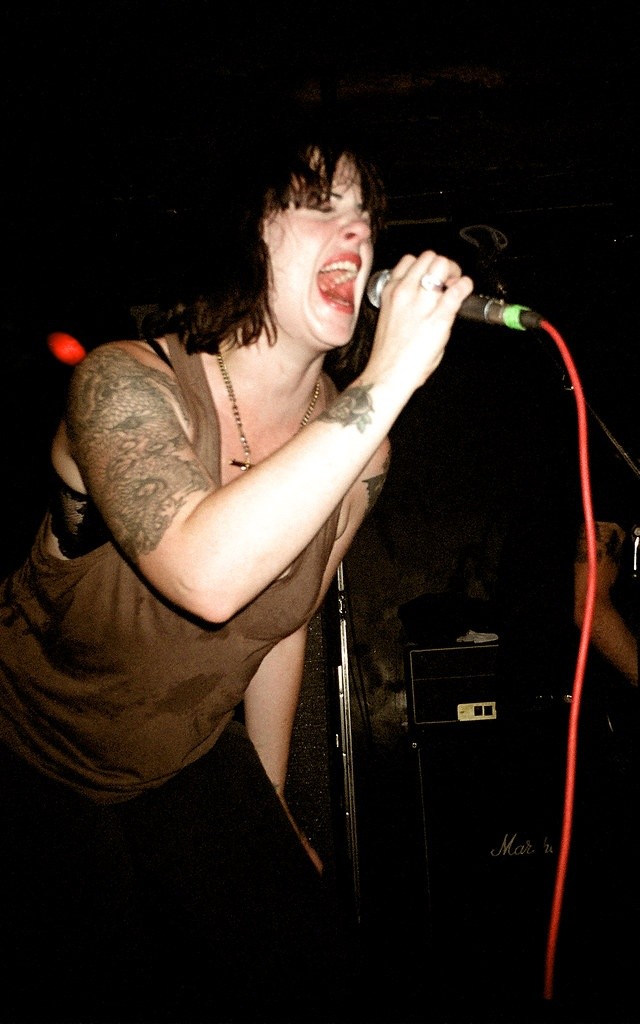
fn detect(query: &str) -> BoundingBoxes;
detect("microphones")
[369,270,541,331]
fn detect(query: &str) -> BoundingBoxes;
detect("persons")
[573,446,640,824]
[0,111,475,1024]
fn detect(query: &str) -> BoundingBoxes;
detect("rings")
[419,274,446,291]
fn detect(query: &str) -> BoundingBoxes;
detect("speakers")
[405,715,565,964]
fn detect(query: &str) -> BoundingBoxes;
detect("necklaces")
[214,352,320,471]
